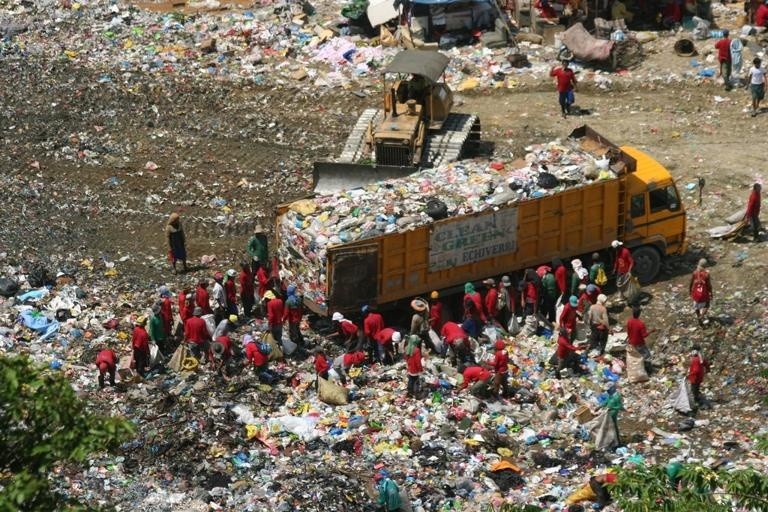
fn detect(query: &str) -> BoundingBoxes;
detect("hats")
[135,316,148,327]
[517,280,527,293]
[211,342,225,355]
[431,290,438,299]
[254,225,266,234]
[261,290,276,302]
[100,363,107,374]
[215,269,237,281]
[182,283,191,293]
[332,312,344,320]
[482,276,511,287]
[411,299,425,312]
[611,239,623,249]
[569,295,578,308]
[151,304,161,315]
[229,314,238,326]
[252,256,260,263]
[191,307,202,317]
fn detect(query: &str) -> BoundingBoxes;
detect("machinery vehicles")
[308,49,484,199]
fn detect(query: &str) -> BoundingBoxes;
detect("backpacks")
[252,340,272,354]
[594,263,608,286]
[496,292,505,312]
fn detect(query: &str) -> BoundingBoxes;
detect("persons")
[687,340,711,403]
[246,225,269,279]
[549,60,578,117]
[165,213,190,275]
[315,241,648,402]
[745,182,762,242]
[404,74,433,108]
[688,257,715,327]
[714,30,732,93]
[532,1,768,34]
[130,258,304,384]
[427,4,451,41]
[94,348,118,387]
[744,58,767,117]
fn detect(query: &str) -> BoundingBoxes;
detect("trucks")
[272,124,690,334]
